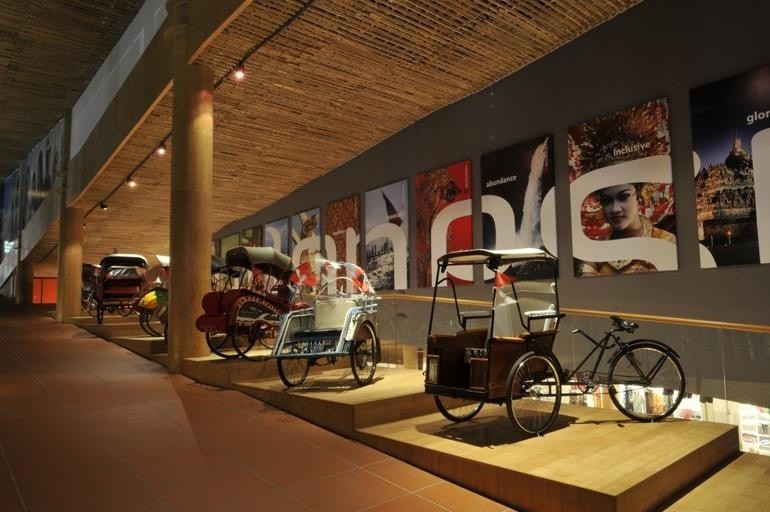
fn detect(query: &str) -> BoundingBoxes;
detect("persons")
[575,182,678,277]
[7,132,64,241]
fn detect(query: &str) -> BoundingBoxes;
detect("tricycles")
[425,248,686,437]
[82,254,169,346]
[196,247,314,358]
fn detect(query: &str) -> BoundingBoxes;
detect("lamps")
[158,144,167,155]
[234,61,245,82]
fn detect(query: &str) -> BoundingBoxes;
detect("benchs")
[289,297,359,339]
[104,278,141,296]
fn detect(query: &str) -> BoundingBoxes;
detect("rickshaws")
[270,259,382,387]
